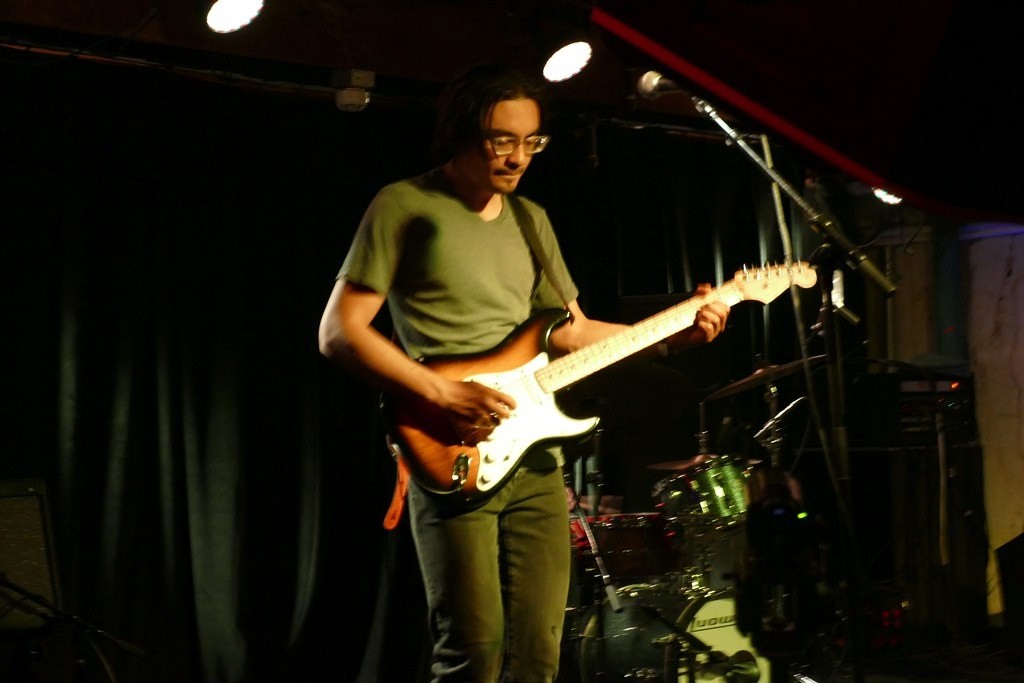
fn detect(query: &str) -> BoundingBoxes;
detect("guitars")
[377,260,819,505]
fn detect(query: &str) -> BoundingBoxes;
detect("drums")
[652,454,755,542]
[580,582,770,683]
[566,511,661,563]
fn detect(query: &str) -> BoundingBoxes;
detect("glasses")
[485,134,550,157]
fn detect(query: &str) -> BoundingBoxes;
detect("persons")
[316,74,731,682]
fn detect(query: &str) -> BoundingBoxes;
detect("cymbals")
[651,454,760,472]
[714,352,832,397]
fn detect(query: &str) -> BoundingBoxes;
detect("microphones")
[641,72,696,107]
